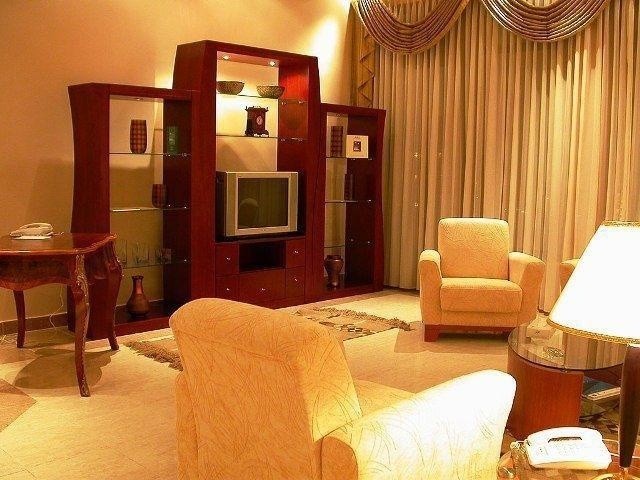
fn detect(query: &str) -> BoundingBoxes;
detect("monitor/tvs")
[217,171,300,239]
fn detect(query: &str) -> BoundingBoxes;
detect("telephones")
[9,223,53,237]
[523,427,612,471]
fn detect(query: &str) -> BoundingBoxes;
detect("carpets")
[123,307,412,372]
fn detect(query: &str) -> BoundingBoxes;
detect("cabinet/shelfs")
[68,40,386,341]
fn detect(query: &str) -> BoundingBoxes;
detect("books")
[581,376,621,401]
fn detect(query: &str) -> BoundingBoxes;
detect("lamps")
[546,220,640,480]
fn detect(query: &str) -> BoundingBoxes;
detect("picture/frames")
[346,135,368,158]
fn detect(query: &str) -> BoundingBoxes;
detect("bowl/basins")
[216,81,244,94]
[256,85,285,98]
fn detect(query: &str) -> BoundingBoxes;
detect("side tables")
[508,317,627,444]
[497,439,640,480]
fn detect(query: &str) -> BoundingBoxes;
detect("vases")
[126,274,150,317]
[330,126,343,157]
[130,120,147,153]
[166,126,179,154]
[324,253,344,290]
[152,184,168,208]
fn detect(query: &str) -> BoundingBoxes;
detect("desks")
[0,234,123,397]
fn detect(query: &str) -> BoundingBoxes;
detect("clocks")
[245,106,269,137]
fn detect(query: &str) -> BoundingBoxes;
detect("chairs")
[418,217,546,342]
[169,297,516,480]
[559,259,579,292]
